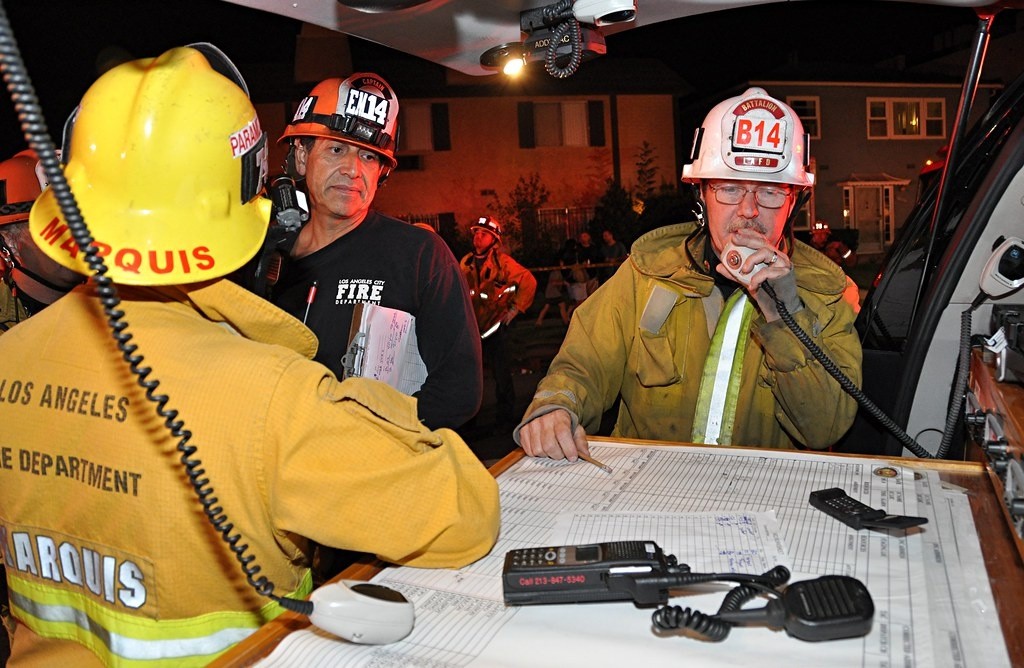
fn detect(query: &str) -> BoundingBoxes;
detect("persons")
[810,221,858,272]
[459,217,537,437]
[0,43,500,668]
[535,231,625,334]
[229,71,483,429]
[513,88,863,462]
[0,150,87,336]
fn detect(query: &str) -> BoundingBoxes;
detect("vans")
[815,72,1024,462]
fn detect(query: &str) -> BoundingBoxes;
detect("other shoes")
[490,419,513,440]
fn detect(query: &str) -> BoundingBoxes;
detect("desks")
[203,435,1024,668]
[970,347,1024,562]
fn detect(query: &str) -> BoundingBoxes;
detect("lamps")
[499,48,525,76]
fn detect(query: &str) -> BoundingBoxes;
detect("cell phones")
[809,487,928,531]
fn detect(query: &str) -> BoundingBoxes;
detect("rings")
[763,251,777,265]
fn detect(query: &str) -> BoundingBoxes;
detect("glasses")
[706,182,791,209]
[276,72,400,178]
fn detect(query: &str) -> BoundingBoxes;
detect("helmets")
[471,216,502,247]
[0,149,63,224]
[681,87,815,187]
[30,46,272,286]
